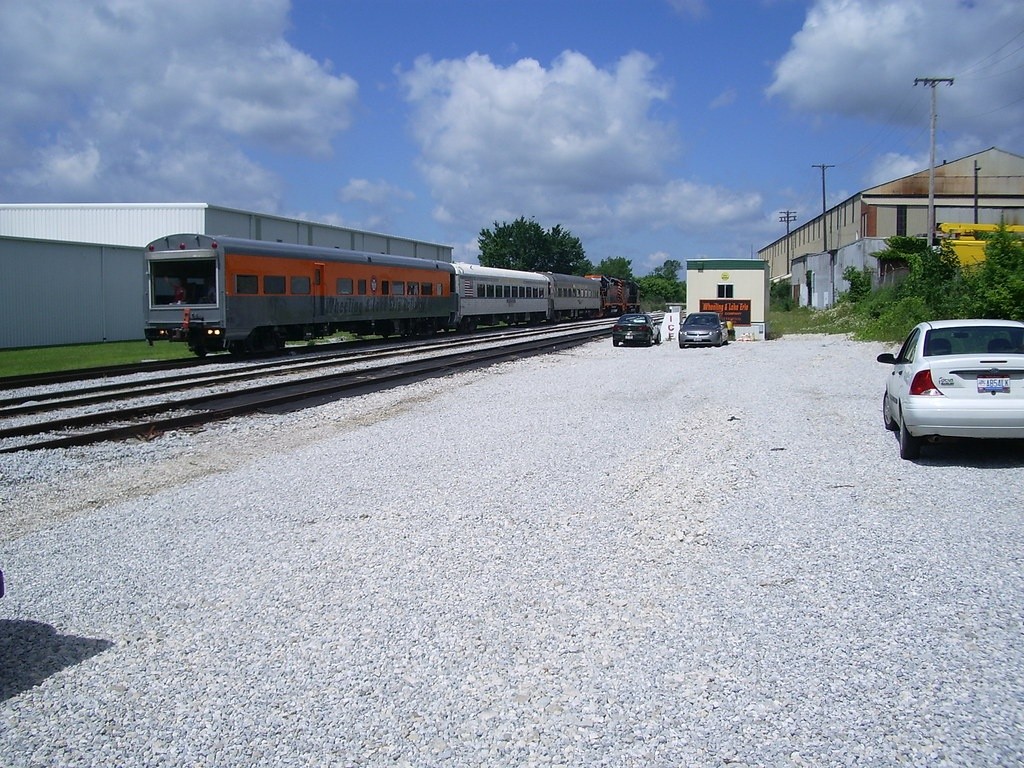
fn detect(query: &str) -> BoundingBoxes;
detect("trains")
[143,232,641,359]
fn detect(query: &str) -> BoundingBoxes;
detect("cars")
[877,319,1024,459]
[678,312,729,349]
[612,314,661,347]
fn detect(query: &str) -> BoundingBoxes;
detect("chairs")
[929,339,952,354]
[988,338,1011,353]
